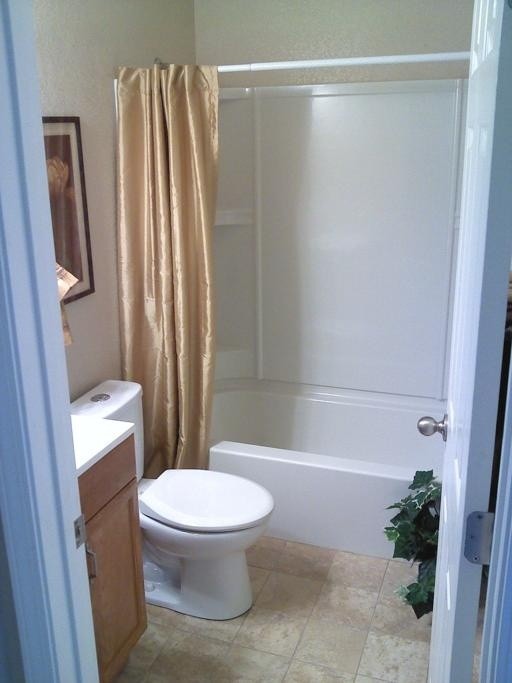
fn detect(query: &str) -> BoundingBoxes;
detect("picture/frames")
[43,116,95,306]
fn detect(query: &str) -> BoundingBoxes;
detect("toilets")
[70,378,274,622]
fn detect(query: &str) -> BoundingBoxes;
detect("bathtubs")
[208,379,446,564]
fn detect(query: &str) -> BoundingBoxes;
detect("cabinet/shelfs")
[78,432,148,683]
